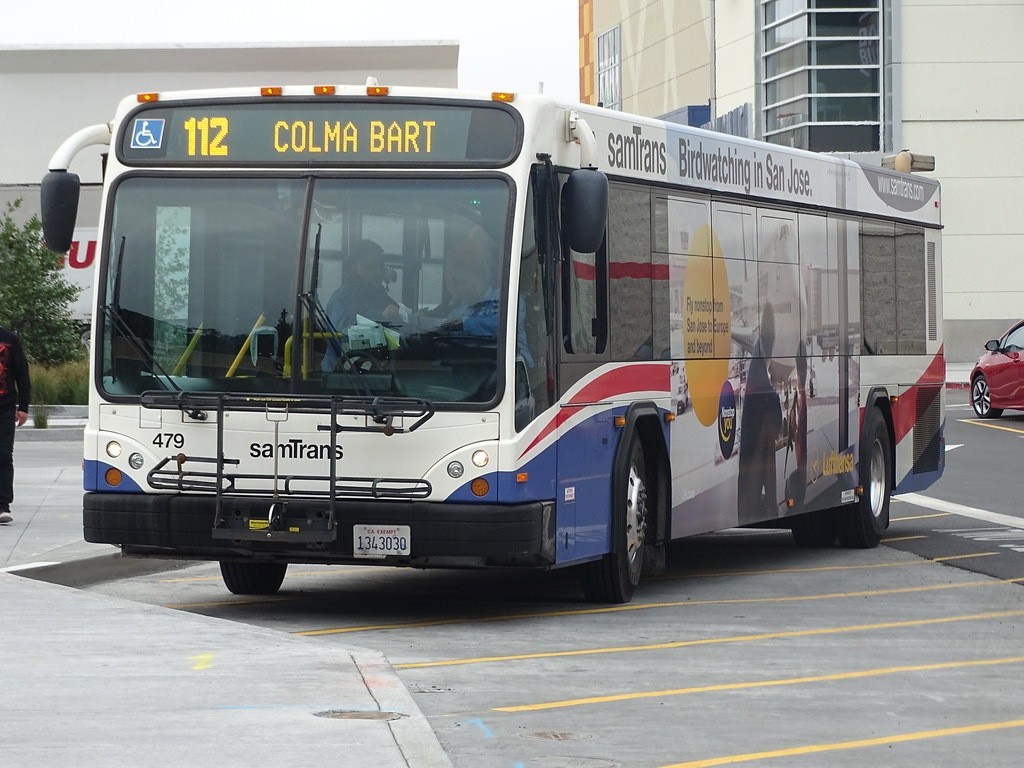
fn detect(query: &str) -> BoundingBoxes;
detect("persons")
[0,323,31,524]
[321,240,531,375]
[738,305,807,527]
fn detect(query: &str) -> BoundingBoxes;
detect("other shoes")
[0,504,14,522]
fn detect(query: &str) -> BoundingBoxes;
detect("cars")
[969,318,1024,419]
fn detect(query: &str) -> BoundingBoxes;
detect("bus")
[40,79,947,604]
